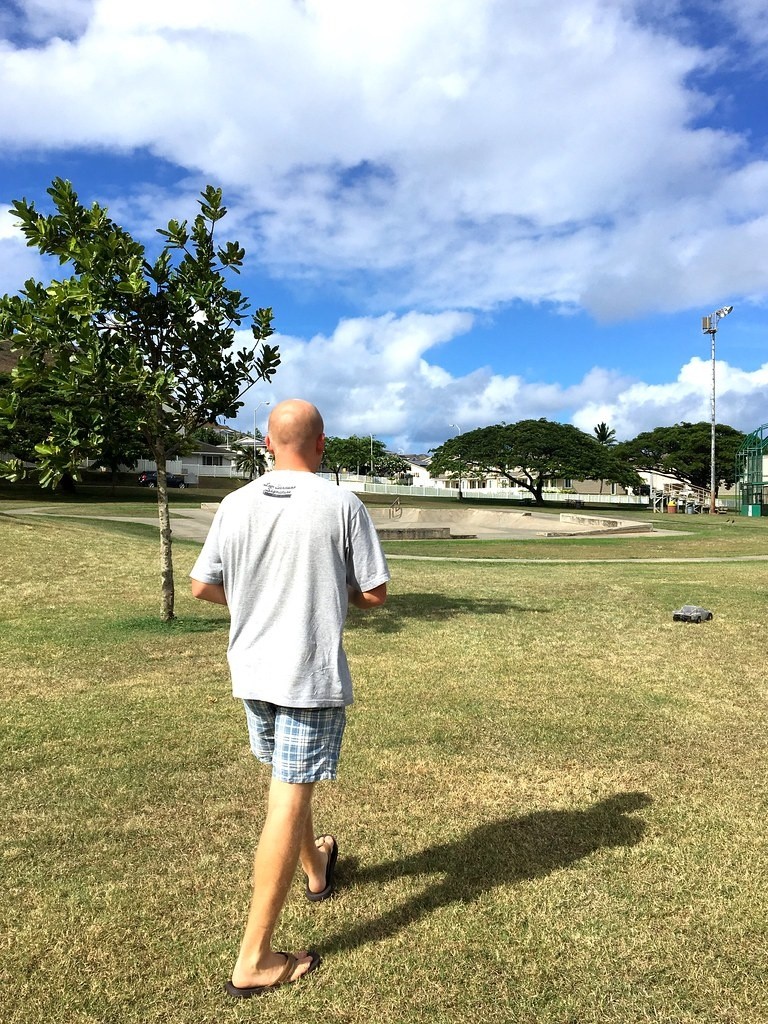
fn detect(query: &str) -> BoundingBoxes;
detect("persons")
[186,399,393,997]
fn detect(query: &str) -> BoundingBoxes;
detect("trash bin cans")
[686,501,694,514]
[668,501,677,513]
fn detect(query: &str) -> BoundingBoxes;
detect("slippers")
[306,835,338,901]
[226,951,320,999]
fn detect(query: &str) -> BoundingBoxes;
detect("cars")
[673,605,713,624]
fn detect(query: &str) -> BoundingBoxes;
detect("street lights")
[253,401,270,480]
[369,434,373,482]
[449,423,462,500]
[702,306,734,513]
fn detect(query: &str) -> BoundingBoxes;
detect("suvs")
[138,471,185,489]
